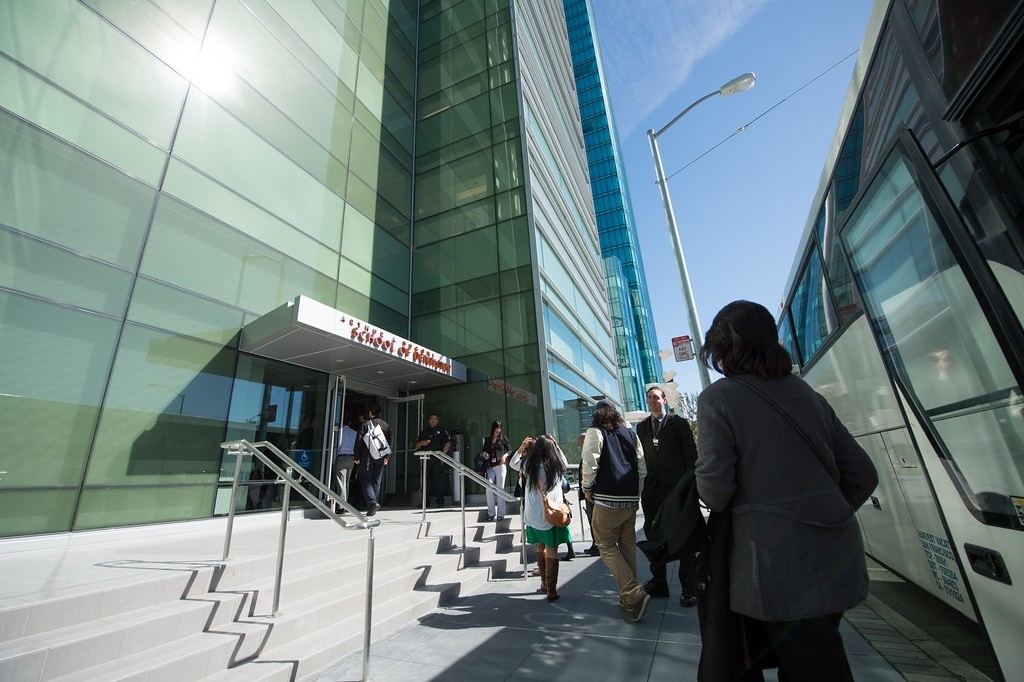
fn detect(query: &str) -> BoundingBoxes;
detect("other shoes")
[533,569,540,576]
[640,580,670,598]
[561,553,575,561]
[627,597,654,612]
[366,502,381,516]
[497,516,503,521]
[337,509,346,514]
[679,589,698,608]
[488,515,495,520]
[629,594,651,623]
[417,502,430,509]
[584,544,600,556]
[438,502,444,507]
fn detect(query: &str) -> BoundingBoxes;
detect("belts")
[338,454,355,456]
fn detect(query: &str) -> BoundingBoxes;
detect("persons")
[578,399,650,622]
[917,325,1024,496]
[474,420,512,521]
[636,387,698,608]
[415,413,450,509]
[696,301,880,682]
[507,434,576,603]
[333,404,392,516]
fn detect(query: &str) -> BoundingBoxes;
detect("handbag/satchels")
[362,419,393,471]
[543,499,572,528]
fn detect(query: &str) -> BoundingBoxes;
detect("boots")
[537,552,547,594]
[546,558,559,602]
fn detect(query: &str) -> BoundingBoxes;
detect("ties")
[653,418,659,435]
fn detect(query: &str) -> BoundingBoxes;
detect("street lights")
[645,71,756,391]
